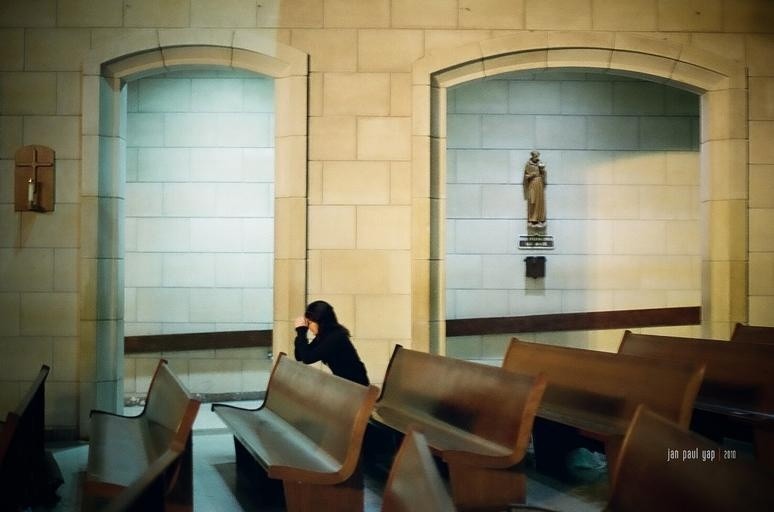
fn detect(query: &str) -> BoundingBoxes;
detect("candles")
[28,178,34,201]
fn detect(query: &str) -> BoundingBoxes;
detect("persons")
[521,149,548,223]
[292,299,368,385]
